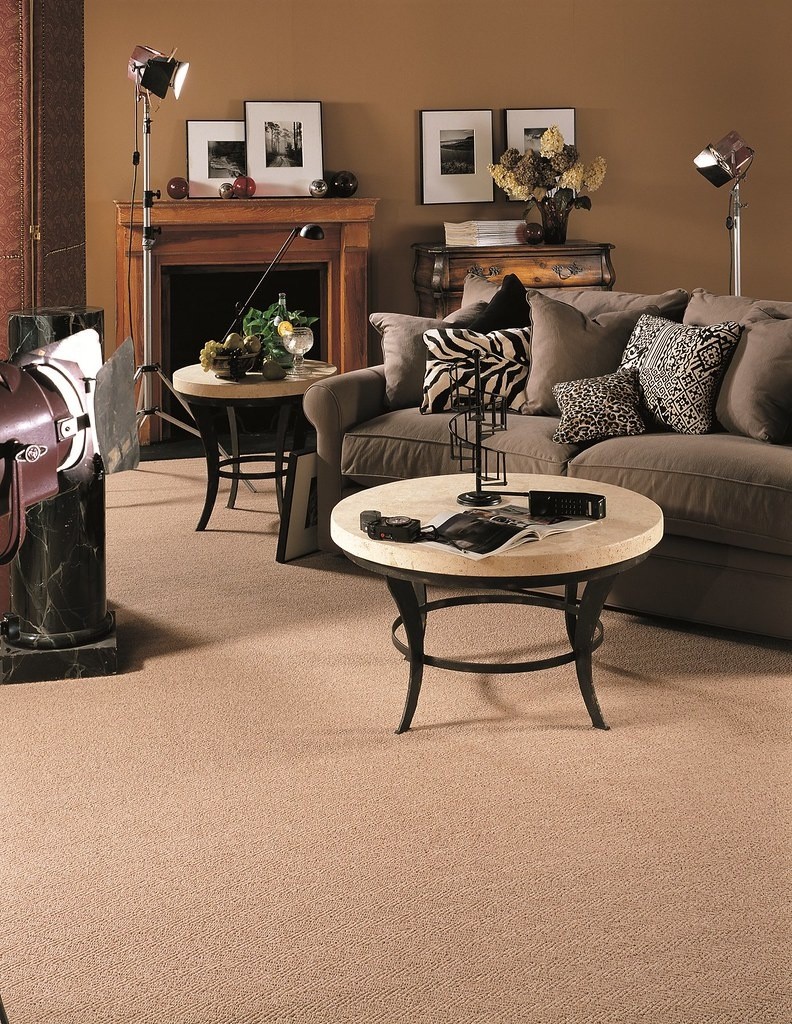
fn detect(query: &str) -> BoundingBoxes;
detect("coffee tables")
[330,472,665,733]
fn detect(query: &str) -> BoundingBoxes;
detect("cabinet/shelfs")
[411,238,617,320]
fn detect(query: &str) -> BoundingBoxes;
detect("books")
[414,503,598,562]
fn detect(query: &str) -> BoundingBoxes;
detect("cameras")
[367,516,420,542]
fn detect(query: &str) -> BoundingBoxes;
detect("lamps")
[126,44,258,494]
[693,130,755,298]
[220,224,325,343]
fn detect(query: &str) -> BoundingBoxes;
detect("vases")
[536,199,575,245]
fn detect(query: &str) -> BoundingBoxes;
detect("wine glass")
[285,328,311,375]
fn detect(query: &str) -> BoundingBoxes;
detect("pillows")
[368,300,489,411]
[467,273,533,334]
[519,291,660,416]
[553,368,645,444]
[420,328,533,415]
[617,313,741,435]
[717,305,792,444]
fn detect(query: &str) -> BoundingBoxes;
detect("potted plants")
[243,302,319,367]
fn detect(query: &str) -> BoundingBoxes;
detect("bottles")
[272,293,292,368]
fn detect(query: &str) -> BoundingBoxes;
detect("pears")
[262,359,287,378]
[223,333,261,353]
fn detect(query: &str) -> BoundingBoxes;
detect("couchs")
[302,274,792,642]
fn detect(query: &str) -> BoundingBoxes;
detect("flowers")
[487,123,608,216]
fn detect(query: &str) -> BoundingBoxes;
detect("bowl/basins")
[210,352,257,381]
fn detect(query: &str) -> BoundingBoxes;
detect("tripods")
[133,91,258,494]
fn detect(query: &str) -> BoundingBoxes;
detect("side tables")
[172,360,338,531]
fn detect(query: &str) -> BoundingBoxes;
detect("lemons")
[278,321,293,336]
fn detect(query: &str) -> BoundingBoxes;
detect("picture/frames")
[276,446,321,564]
[503,108,576,202]
[243,100,325,198]
[420,109,494,205]
[185,119,246,199]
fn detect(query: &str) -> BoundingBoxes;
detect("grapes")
[199,340,243,383]
